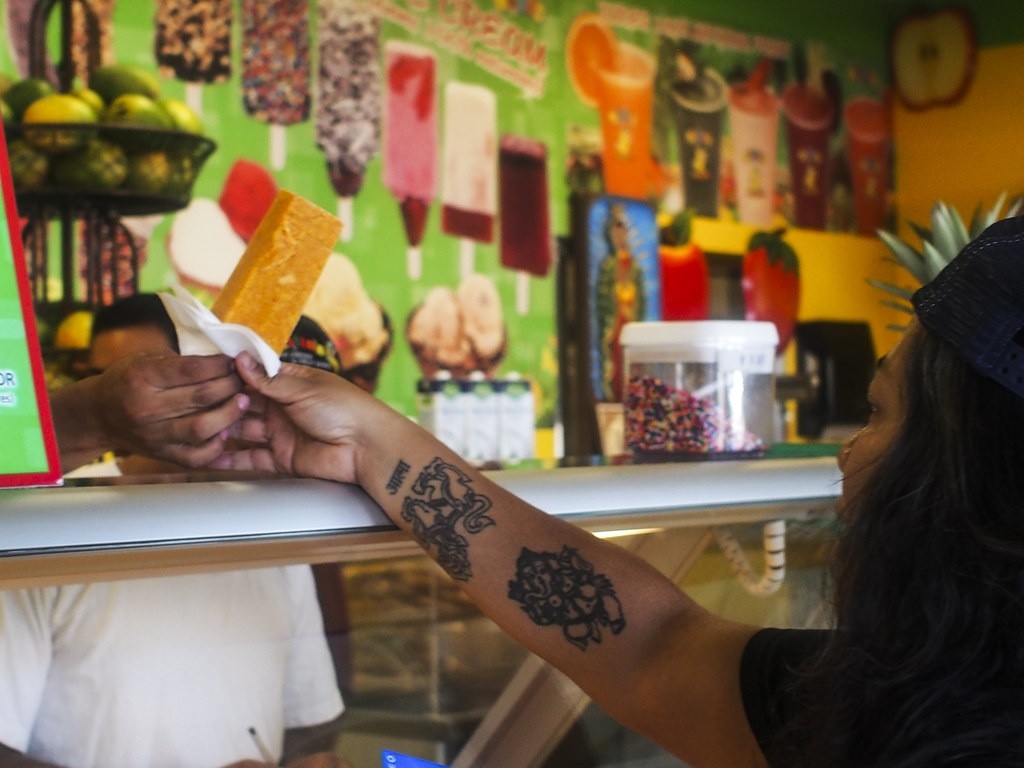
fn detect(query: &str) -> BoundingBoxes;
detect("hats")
[911,215,1024,397]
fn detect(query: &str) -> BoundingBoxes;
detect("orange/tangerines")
[565,12,619,106]
[0,64,202,196]
[33,311,93,393]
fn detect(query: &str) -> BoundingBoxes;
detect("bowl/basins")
[595,402,624,456]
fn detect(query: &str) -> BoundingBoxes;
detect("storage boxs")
[619,320,779,461]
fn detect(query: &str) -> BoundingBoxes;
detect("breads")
[213,189,343,362]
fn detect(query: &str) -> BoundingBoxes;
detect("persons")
[197,214,1024,768]
[0,293,345,767]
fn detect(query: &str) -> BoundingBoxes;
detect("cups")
[590,42,658,199]
[783,95,833,231]
[732,90,780,226]
[665,66,729,220]
[843,96,892,238]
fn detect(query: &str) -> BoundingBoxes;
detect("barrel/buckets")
[618,319,785,457]
